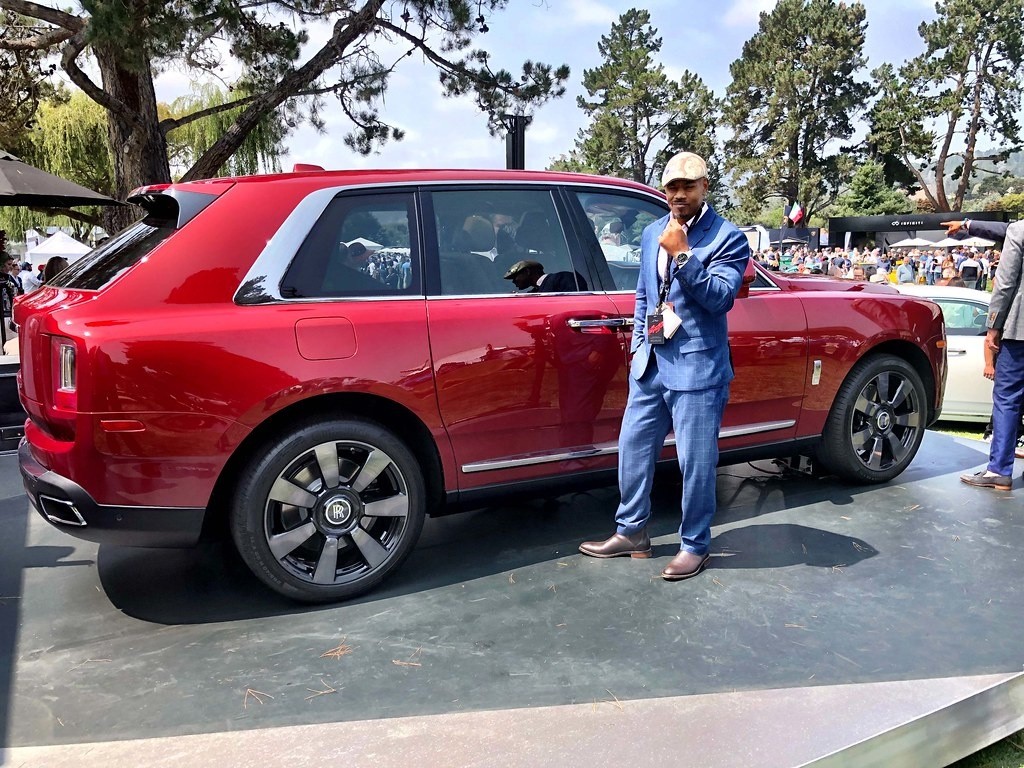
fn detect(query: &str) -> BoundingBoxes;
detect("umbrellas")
[0,149,129,207]
[889,237,997,248]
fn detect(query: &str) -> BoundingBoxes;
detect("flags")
[784,201,803,225]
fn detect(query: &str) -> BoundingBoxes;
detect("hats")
[662,152,708,187]
[22,262,33,268]
[503,260,543,280]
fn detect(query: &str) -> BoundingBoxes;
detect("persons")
[588,219,641,261]
[0,250,71,356]
[938,214,1024,491]
[503,260,589,292]
[337,243,412,290]
[752,243,1002,291]
[577,152,749,581]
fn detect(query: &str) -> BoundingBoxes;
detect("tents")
[26,231,94,278]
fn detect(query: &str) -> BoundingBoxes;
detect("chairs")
[502,208,552,287]
[462,214,498,292]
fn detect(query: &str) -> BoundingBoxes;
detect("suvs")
[11,163,952,607]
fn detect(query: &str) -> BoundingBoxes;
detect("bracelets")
[961,218,969,230]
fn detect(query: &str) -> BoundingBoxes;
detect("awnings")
[340,237,383,251]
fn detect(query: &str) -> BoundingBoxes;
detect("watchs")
[673,251,693,266]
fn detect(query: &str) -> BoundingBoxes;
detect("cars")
[893,283,1007,422]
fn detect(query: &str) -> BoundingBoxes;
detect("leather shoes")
[579,525,652,558]
[961,469,1013,490]
[662,550,710,579]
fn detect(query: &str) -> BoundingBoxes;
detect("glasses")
[14,268,19,271]
